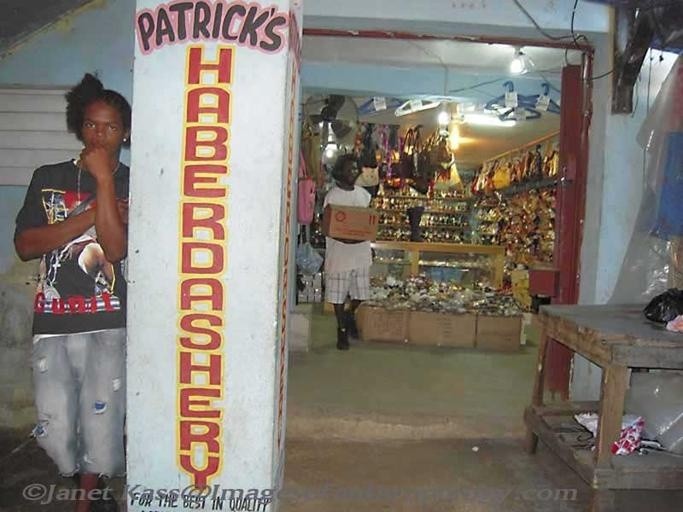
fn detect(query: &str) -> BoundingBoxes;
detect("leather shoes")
[472,195,503,246]
[370,182,468,244]
[370,255,493,269]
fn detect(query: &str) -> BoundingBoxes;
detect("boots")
[407,206,427,242]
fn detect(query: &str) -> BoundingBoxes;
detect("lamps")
[509,45,532,76]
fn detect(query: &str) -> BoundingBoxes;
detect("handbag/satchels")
[573,410,644,456]
[378,129,456,194]
[297,222,324,275]
[525,145,542,179]
[298,150,315,222]
[543,143,560,178]
[470,157,527,193]
[352,128,377,168]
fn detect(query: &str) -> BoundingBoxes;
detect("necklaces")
[75,154,121,211]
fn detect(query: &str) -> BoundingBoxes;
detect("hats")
[354,166,379,187]
[321,143,340,169]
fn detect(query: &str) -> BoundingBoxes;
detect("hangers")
[394,99,440,117]
[358,97,402,114]
[499,107,541,121]
[484,81,537,109]
[527,83,560,114]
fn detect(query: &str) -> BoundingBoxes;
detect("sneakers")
[73,474,118,512]
[499,183,555,263]
[369,274,521,316]
[337,327,348,349]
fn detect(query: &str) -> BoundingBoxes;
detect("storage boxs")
[323,203,379,241]
[409,311,476,348]
[477,316,522,349]
[355,303,410,344]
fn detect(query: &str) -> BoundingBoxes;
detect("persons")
[15,90,130,507]
[323,155,376,350]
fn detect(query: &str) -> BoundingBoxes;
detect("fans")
[304,93,359,144]
